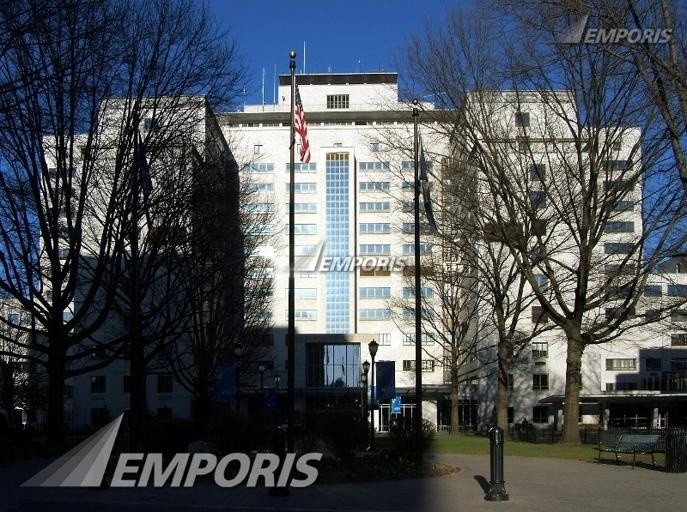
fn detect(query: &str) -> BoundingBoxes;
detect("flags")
[417,125,439,235]
[294,76,310,164]
[138,132,153,200]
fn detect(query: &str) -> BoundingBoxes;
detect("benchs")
[512,424,533,432]
[592,435,660,471]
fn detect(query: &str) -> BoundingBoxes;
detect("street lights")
[272,372,283,410]
[359,339,381,436]
[257,363,268,417]
[233,342,245,417]
